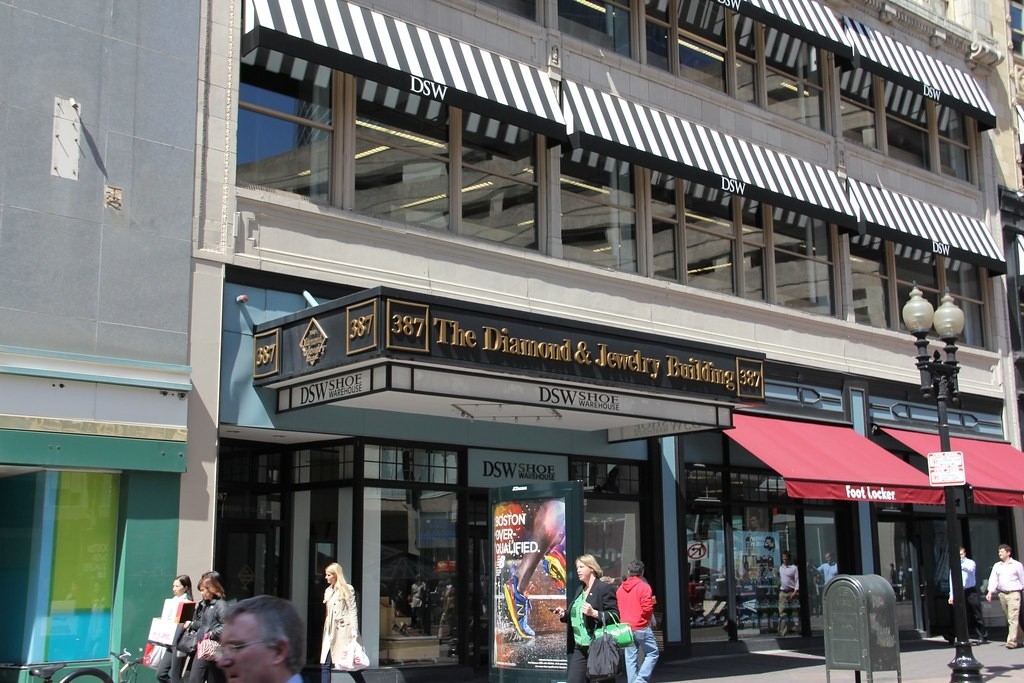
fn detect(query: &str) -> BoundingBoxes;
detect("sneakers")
[542,544,566,596]
[504,577,535,638]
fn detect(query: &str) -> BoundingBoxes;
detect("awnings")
[415,519,455,549]
[879,428,1024,507]
[721,414,945,505]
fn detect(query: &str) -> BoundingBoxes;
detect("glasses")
[215,635,278,661]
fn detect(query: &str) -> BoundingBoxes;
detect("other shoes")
[1005,641,1023,648]
[978,636,987,643]
[942,632,955,644]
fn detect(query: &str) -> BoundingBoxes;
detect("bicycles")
[0,661,117,683]
[111,648,145,683]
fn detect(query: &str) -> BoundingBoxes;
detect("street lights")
[901,280,996,683]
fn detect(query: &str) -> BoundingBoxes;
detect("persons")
[503,499,566,639]
[777,550,800,637]
[747,514,765,532]
[553,554,660,683]
[380,572,457,645]
[942,547,987,644]
[986,544,1024,648]
[806,552,907,616]
[143,563,367,683]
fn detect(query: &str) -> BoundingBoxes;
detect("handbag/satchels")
[335,637,370,671]
[586,610,634,681]
[176,621,198,652]
[197,631,223,662]
[142,641,167,671]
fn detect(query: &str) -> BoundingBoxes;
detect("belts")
[780,590,799,593]
[1000,590,1020,595]
